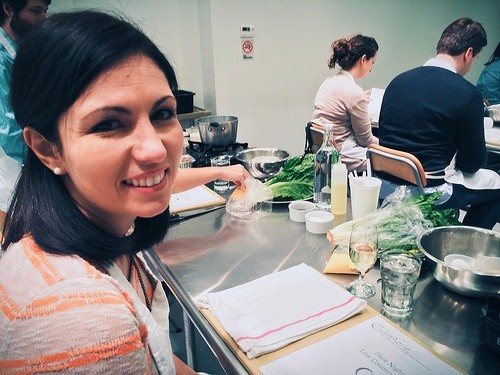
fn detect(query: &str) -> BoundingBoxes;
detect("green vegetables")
[262,150,340,200]
[326,190,465,254]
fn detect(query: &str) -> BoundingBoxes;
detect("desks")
[370,116,500,156]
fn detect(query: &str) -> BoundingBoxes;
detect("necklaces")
[126,252,152,312]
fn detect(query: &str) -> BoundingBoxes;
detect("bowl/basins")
[486,104,500,121]
[236,147,291,179]
[416,225,500,298]
[305,209,335,235]
[288,201,316,222]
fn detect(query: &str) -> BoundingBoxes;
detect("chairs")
[366,144,472,221]
[305,122,368,178]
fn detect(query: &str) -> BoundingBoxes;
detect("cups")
[349,174,382,220]
[212,155,231,190]
[380,247,422,320]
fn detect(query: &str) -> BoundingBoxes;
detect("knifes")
[169,204,227,222]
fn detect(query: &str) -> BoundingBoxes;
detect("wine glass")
[343,223,379,298]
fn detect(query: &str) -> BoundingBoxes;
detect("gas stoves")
[186,139,249,166]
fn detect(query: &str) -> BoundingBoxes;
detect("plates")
[265,195,313,203]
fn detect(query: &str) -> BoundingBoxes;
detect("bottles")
[329,156,348,216]
[314,123,343,212]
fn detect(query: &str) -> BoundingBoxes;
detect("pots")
[196,115,239,145]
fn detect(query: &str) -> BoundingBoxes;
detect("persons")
[375,18,500,230]
[0,10,197,375]
[312,34,379,174]
[0,0,250,247]
[475,40,500,117]
